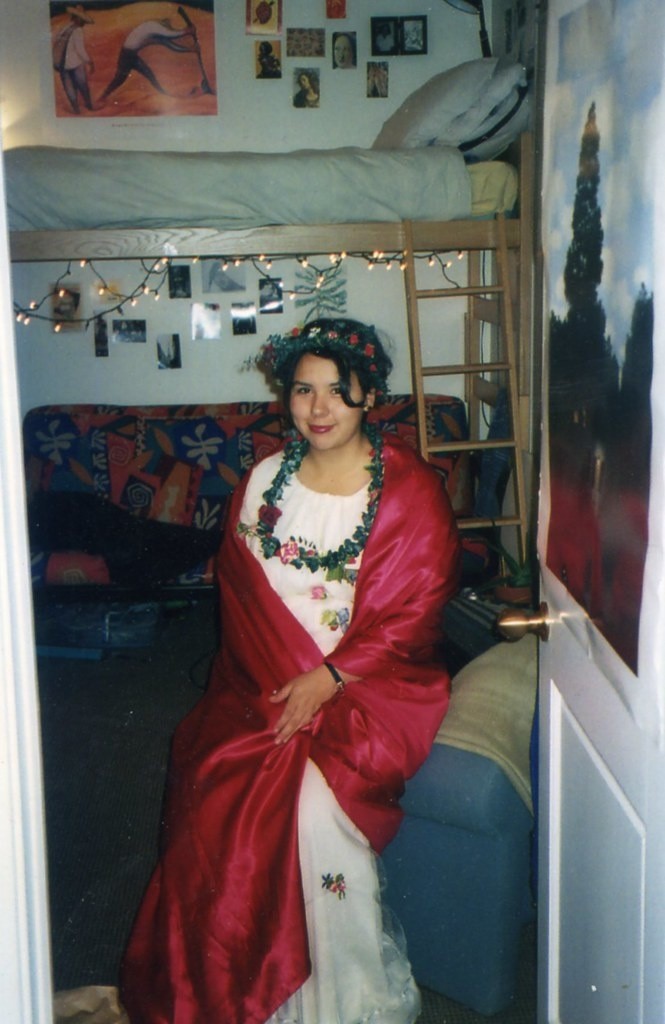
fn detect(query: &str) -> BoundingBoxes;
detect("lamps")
[443,0,492,57]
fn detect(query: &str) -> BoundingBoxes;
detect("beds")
[3,132,535,1018]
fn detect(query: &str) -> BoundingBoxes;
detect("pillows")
[369,56,533,164]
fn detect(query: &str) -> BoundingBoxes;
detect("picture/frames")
[371,15,427,56]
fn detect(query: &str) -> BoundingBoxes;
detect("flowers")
[243,325,392,407]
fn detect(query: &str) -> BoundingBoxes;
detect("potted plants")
[465,532,534,604]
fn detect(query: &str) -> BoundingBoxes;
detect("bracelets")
[325,659,344,689]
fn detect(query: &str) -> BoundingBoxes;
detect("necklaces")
[258,422,384,570]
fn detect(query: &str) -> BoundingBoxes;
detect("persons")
[119,319,461,1024]
